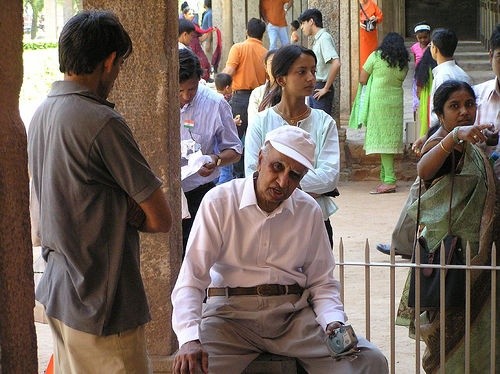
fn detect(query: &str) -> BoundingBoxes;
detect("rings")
[473,135,478,139]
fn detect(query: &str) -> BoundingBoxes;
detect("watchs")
[214,153,221,166]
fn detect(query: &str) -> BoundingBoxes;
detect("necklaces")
[463,144,486,178]
[276,104,309,124]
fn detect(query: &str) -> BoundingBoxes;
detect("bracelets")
[440,141,452,154]
[454,126,464,145]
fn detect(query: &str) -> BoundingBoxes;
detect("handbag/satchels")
[408,235,466,306]
[349,82,362,129]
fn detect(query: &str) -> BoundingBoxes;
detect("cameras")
[366,19,376,31]
[324,325,358,359]
[483,123,499,146]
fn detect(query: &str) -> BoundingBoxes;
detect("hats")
[265,124,317,175]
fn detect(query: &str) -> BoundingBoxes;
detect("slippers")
[370,187,396,194]
[375,183,382,188]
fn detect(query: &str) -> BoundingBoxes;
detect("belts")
[207,284,300,296]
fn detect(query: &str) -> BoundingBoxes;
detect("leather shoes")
[376,243,411,259]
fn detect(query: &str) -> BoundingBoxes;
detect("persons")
[173,126,390,374]
[248,49,309,120]
[412,26,500,155]
[429,32,473,128]
[26,10,172,374]
[360,32,410,193]
[179,0,270,184]
[359,0,383,71]
[396,83,500,374]
[290,20,300,45]
[244,45,340,249]
[179,47,242,252]
[298,8,341,116]
[404,23,438,147]
[257,0,294,51]
